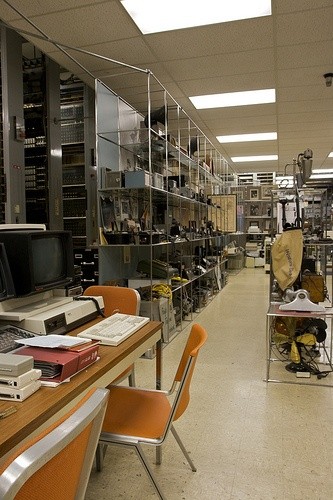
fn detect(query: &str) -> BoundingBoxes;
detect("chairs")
[95,321,208,500]
[0,386,111,500]
[81,285,142,389]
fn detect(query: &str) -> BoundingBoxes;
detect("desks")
[0,316,165,500]
[261,301,333,388]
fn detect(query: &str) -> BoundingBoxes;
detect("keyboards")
[0,324,40,354]
[77,312,150,346]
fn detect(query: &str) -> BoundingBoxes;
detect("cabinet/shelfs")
[0,22,333,359]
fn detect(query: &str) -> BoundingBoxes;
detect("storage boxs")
[244,256,255,267]
[139,231,163,245]
[0,368,42,391]
[227,248,237,255]
[0,379,41,402]
[0,352,34,378]
[254,257,264,267]
[168,295,177,337]
[79,266,99,282]
[153,172,164,190]
[128,275,172,299]
[106,171,120,188]
[103,230,134,244]
[140,298,169,341]
[124,170,153,186]
[73,248,99,266]
[301,274,325,302]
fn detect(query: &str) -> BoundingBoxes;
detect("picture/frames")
[250,188,260,200]
[206,193,237,233]
[251,221,259,228]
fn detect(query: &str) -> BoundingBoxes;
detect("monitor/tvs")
[0,230,75,302]
